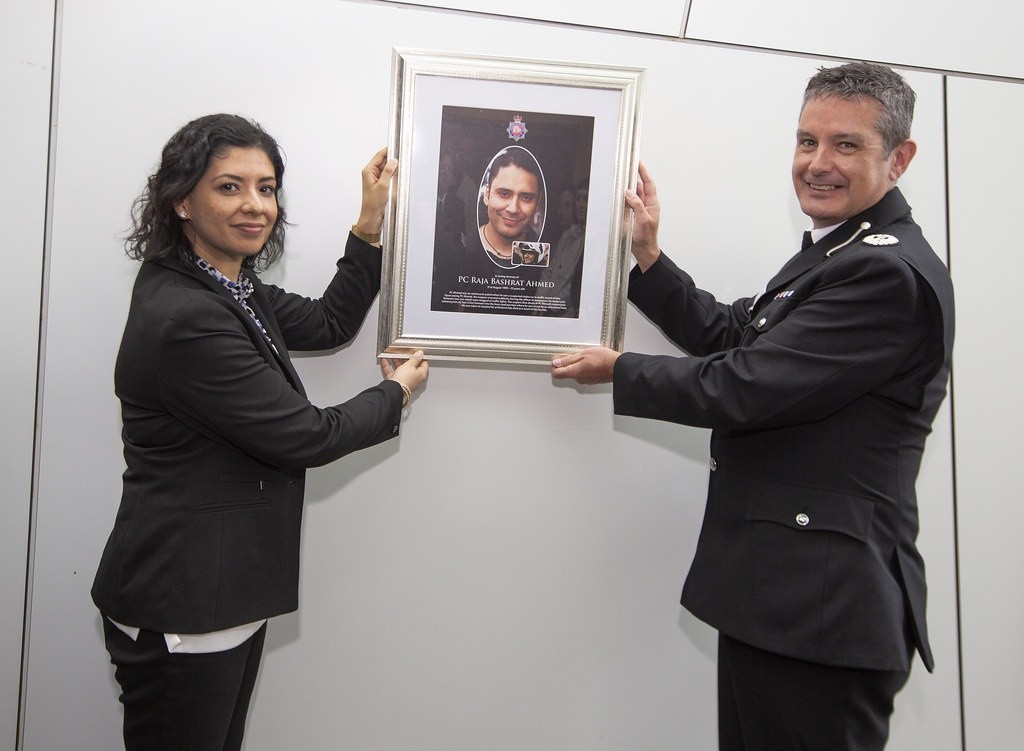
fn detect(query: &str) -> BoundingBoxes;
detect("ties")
[800,231,814,253]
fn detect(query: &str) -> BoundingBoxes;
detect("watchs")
[352,224,382,244]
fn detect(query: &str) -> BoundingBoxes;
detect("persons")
[90,113,429,751]
[438,145,588,318]
[551,61,956,751]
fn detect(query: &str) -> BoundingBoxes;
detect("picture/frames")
[385,49,648,367]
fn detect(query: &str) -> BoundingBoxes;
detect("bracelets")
[389,379,412,409]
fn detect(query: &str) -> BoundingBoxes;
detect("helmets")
[521,243,543,254]
